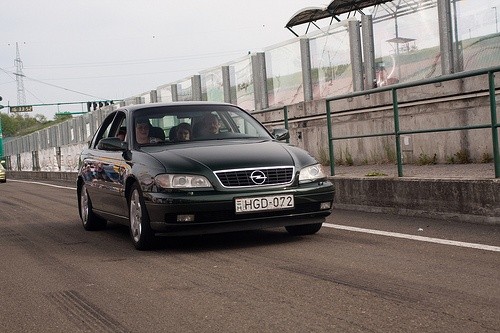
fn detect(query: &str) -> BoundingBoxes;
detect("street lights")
[492,6,498,32]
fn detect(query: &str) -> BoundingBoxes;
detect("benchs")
[153,124,178,139]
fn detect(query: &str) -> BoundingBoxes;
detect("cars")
[0,159,7,183]
[75,100,336,249]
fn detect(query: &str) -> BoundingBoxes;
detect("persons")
[203,114,221,134]
[176,122,192,142]
[136,118,160,144]
